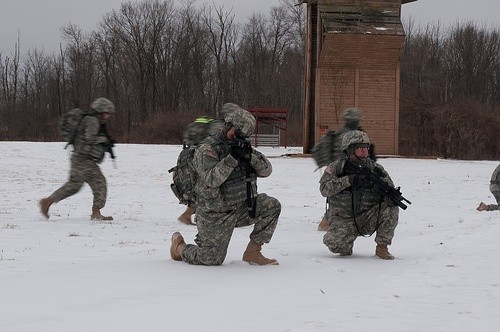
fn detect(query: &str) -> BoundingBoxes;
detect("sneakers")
[170,231,184,261]
[242,243,279,265]
[376,246,395,260]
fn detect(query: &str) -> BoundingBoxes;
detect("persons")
[39,96,116,221]
[170,102,281,266]
[476,164,500,211]
[317,107,377,232]
[319,129,404,261]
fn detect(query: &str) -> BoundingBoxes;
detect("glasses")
[357,145,370,151]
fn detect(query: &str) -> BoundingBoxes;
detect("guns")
[98,121,117,160]
[342,159,412,211]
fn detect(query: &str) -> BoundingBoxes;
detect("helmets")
[341,130,371,152]
[90,96,115,114]
[342,107,364,122]
[220,103,241,120]
[224,109,256,138]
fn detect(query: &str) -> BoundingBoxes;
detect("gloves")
[349,174,366,186]
[230,140,254,161]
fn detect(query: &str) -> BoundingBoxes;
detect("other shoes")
[178,207,195,225]
[477,202,487,211]
[40,198,51,219]
[90,208,114,221]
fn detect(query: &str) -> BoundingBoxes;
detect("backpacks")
[312,129,340,168]
[170,147,197,206]
[56,108,87,145]
[181,117,218,147]
